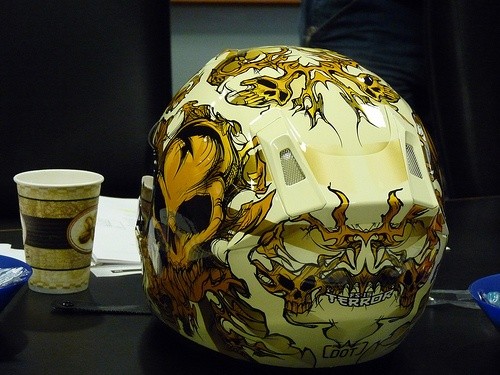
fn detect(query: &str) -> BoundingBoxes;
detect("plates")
[0,253,33,313]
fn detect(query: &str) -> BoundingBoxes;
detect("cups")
[12,168,104,294]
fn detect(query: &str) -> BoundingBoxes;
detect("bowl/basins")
[468,272,500,328]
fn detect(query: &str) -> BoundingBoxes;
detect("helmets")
[136,45,450,375]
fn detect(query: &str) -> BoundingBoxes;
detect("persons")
[299,0,423,122]
